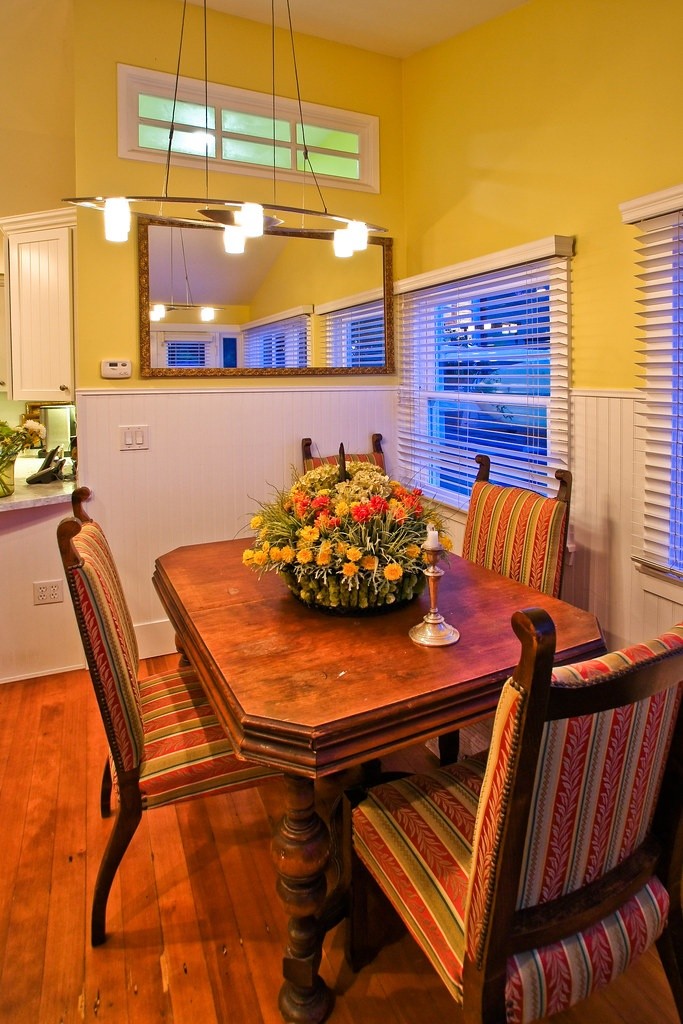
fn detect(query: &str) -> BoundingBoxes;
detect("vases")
[278,563,427,618]
[0,453,18,497]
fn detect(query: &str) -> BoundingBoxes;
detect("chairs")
[439,454,573,766]
[341,606,683,1024]
[57,486,382,948]
[302,433,387,476]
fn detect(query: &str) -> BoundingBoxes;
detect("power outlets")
[32,579,64,605]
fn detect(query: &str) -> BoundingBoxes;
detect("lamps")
[61,0,390,257]
[149,227,227,322]
[150,227,226,322]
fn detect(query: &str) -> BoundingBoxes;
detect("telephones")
[24,445,66,486]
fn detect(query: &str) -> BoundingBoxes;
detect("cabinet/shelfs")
[0,205,76,402]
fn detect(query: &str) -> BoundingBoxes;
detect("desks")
[153,536,610,1024]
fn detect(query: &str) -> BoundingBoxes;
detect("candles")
[426,522,438,548]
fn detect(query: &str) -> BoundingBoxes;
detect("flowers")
[233,461,467,609]
[0,419,47,493]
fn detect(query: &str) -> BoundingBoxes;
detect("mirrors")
[138,215,396,377]
